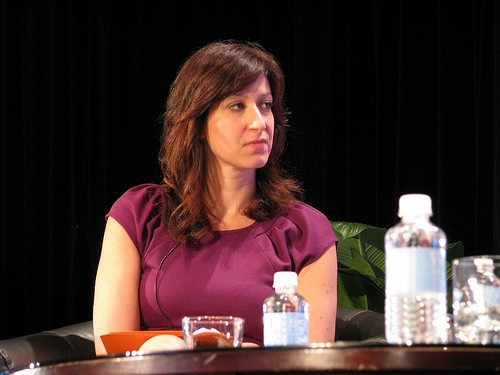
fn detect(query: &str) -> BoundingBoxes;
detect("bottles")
[385,193,448,345]
[263,272,309,347]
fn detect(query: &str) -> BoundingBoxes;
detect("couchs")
[0,306,390,375]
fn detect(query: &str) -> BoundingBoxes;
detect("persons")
[92,40,339,356]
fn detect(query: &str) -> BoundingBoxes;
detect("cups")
[451,255,500,345]
[182,316,244,349]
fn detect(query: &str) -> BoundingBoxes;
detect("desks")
[8,341,500,375]
